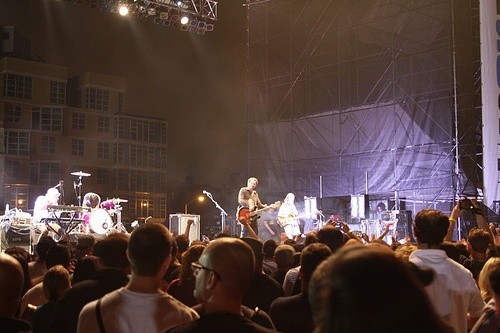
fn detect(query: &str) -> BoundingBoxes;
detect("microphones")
[203,190,212,196]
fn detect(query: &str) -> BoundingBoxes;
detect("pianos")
[39,205,90,242]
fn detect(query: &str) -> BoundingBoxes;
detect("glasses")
[191,261,222,283]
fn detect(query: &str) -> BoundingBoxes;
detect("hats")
[408,261,435,287]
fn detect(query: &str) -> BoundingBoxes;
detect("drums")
[102,200,116,215]
[83,208,112,234]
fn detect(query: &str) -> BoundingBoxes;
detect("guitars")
[238,201,281,226]
[276,211,322,228]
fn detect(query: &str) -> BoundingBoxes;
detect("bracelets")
[254,306,260,319]
[449,218,456,222]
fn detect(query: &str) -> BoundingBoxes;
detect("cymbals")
[70,172,92,176]
[114,199,128,202]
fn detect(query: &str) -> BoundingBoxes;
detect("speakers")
[394,209,412,242]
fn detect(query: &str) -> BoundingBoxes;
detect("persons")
[0,197,500,333]
[33,188,60,220]
[278,193,301,239]
[238,177,276,238]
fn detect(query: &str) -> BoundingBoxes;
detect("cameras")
[459,199,475,212]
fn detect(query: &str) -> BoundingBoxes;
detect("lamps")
[110,0,213,35]
